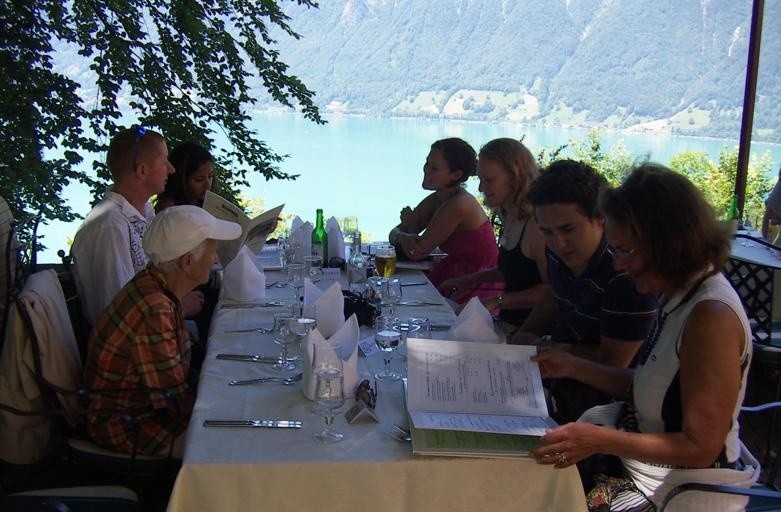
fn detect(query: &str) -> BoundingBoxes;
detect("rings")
[409,248,418,256]
[556,453,569,466]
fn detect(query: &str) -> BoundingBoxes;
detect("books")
[202,189,286,273]
[408,337,563,459]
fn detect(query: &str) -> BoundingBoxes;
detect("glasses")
[602,241,637,259]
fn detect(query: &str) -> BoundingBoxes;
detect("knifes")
[203,419,305,429]
[215,353,294,363]
[375,245,399,305]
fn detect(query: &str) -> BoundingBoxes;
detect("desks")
[724,229,781,324]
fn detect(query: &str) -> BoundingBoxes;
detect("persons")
[390,137,503,318]
[82,205,242,460]
[762,165,781,238]
[155,143,278,237]
[531,163,754,512]
[438,138,547,335]
[70,124,176,330]
[506,161,659,428]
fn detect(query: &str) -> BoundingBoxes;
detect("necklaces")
[639,267,721,367]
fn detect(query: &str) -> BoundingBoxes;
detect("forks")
[229,372,304,386]
[391,422,413,444]
[223,326,275,335]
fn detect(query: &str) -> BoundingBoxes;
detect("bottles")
[347,231,368,297]
[311,208,328,269]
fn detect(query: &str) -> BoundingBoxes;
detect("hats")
[141,204,243,265]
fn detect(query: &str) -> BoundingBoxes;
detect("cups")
[273,313,296,371]
[374,315,403,383]
[368,276,404,315]
[287,264,305,301]
[312,373,345,443]
[403,316,432,371]
[307,341,347,416]
[338,215,359,243]
[289,302,318,361]
[303,256,322,283]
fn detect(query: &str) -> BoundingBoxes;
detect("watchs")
[498,293,504,307]
[393,229,402,250]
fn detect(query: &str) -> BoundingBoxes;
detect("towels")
[288,215,313,262]
[324,215,346,266]
[301,313,361,402]
[447,294,499,343]
[222,243,267,303]
[302,276,347,338]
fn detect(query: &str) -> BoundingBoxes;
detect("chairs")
[14,269,172,470]
[618,342,781,512]
[1,495,150,512]
[4,201,47,308]
